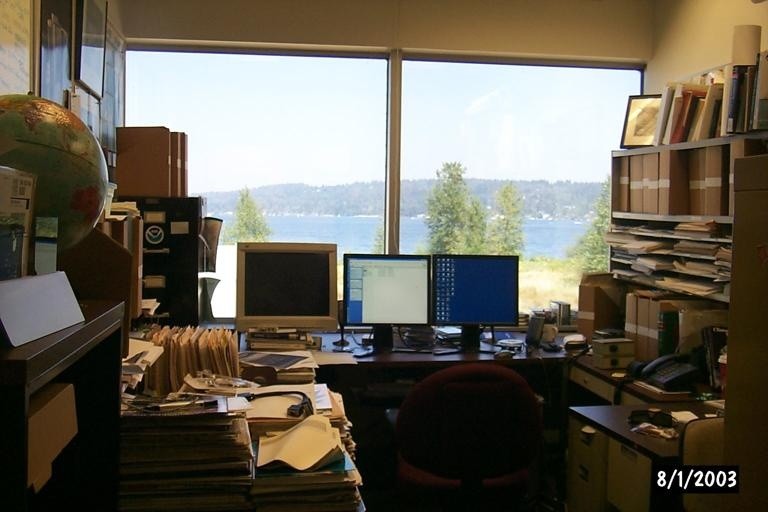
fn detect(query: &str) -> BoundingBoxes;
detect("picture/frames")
[74,0,109,100]
[619,94,662,149]
[69,91,102,142]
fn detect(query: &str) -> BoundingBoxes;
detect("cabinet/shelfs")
[566,361,660,406]
[565,403,725,511]
[0,228,134,512]
[608,128,768,305]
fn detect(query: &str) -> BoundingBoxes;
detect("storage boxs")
[27,382,78,488]
[578,271,624,329]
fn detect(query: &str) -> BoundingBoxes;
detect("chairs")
[682,416,725,511]
[393,363,544,512]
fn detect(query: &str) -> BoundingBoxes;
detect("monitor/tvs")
[343,252,432,357]
[238,242,339,335]
[434,254,519,356]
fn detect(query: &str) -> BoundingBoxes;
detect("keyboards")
[362,379,415,399]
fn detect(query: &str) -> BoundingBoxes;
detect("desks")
[198,324,588,378]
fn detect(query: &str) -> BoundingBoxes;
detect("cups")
[542,325,558,342]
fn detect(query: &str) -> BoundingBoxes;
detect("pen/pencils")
[144,400,218,411]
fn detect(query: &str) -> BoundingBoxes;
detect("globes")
[0,95,109,255]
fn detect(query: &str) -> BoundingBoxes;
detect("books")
[111,123,187,198]
[578,22,768,401]
[120,317,364,511]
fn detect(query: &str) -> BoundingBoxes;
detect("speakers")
[525,316,547,350]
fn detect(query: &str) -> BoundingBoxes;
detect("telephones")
[641,354,701,390]
